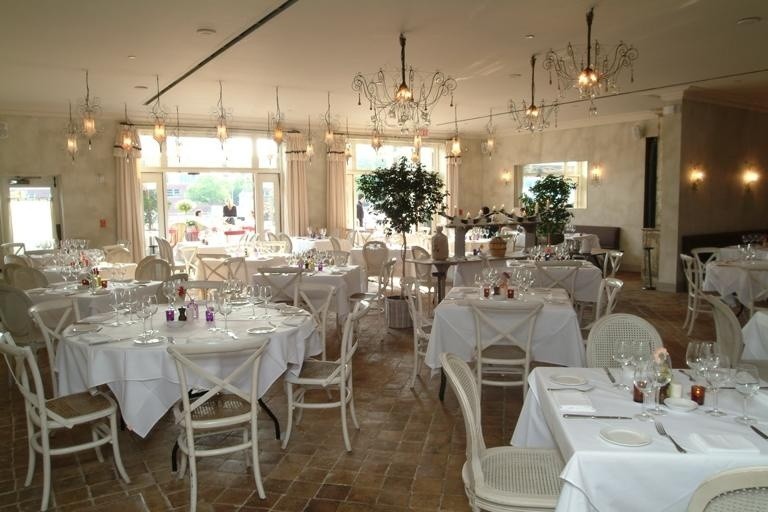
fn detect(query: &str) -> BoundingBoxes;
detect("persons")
[222,199,237,225]
[470,207,499,239]
[357,194,365,227]
[193,210,217,231]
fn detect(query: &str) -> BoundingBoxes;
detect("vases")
[187,302,199,317]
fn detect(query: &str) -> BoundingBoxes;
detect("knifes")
[563,413,633,420]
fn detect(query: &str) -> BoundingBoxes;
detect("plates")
[599,428,651,446]
[665,397,698,411]
[247,326,277,333]
[133,336,165,343]
[551,375,587,386]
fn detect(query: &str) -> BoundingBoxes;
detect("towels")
[548,292,569,301]
[78,333,114,345]
[84,311,114,321]
[695,430,760,454]
[281,314,306,327]
[553,389,595,413]
[444,291,465,299]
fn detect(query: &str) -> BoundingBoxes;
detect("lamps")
[306,115,314,166]
[81,71,100,150]
[352,32,457,133]
[118,101,135,156]
[150,76,171,151]
[487,109,496,157]
[741,167,758,203]
[690,163,716,198]
[324,92,333,154]
[452,105,462,161]
[509,54,558,135]
[544,6,639,117]
[414,126,422,155]
[63,101,80,158]
[213,79,232,151]
[344,119,353,163]
[370,123,382,151]
[272,87,286,153]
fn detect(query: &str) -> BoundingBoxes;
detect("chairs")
[161,238,187,273]
[103,239,134,264]
[535,260,581,310]
[177,281,223,303]
[688,468,767,512]
[747,268,767,320]
[169,222,254,245]
[359,225,434,247]
[156,234,166,260]
[154,272,190,304]
[0,331,131,511]
[134,255,158,281]
[281,300,370,453]
[224,258,251,297]
[240,232,293,254]
[363,242,396,291]
[197,253,232,296]
[181,248,199,279]
[1,243,48,290]
[29,300,83,396]
[410,246,438,307]
[705,296,768,386]
[581,278,624,347]
[473,303,543,407]
[587,313,663,370]
[348,263,389,342]
[167,337,271,512]
[681,254,727,336]
[137,259,170,285]
[602,252,622,309]
[292,279,335,401]
[441,352,565,512]
[401,278,434,389]
[692,247,720,320]
[258,267,305,308]
[0,285,60,401]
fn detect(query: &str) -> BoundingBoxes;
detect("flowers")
[178,287,196,309]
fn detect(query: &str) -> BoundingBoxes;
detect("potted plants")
[356,157,451,330]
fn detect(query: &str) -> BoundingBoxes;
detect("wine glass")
[633,364,655,422]
[321,228,326,239]
[109,290,123,327]
[245,285,259,319]
[685,342,700,378]
[699,341,720,377]
[60,266,71,290]
[633,338,650,379]
[747,243,758,265]
[647,354,672,414]
[56,238,85,253]
[259,285,273,318]
[205,288,221,331]
[467,227,489,241]
[71,265,82,290]
[554,245,569,260]
[136,301,151,336]
[290,252,339,270]
[737,245,746,264]
[614,338,633,391]
[218,297,233,333]
[528,246,543,261]
[142,295,159,332]
[512,268,533,302]
[123,289,137,325]
[702,355,731,416]
[163,281,176,311]
[732,364,759,426]
[475,268,498,298]
[741,231,766,245]
[306,226,312,238]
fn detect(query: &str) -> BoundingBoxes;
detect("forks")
[655,423,687,453]
